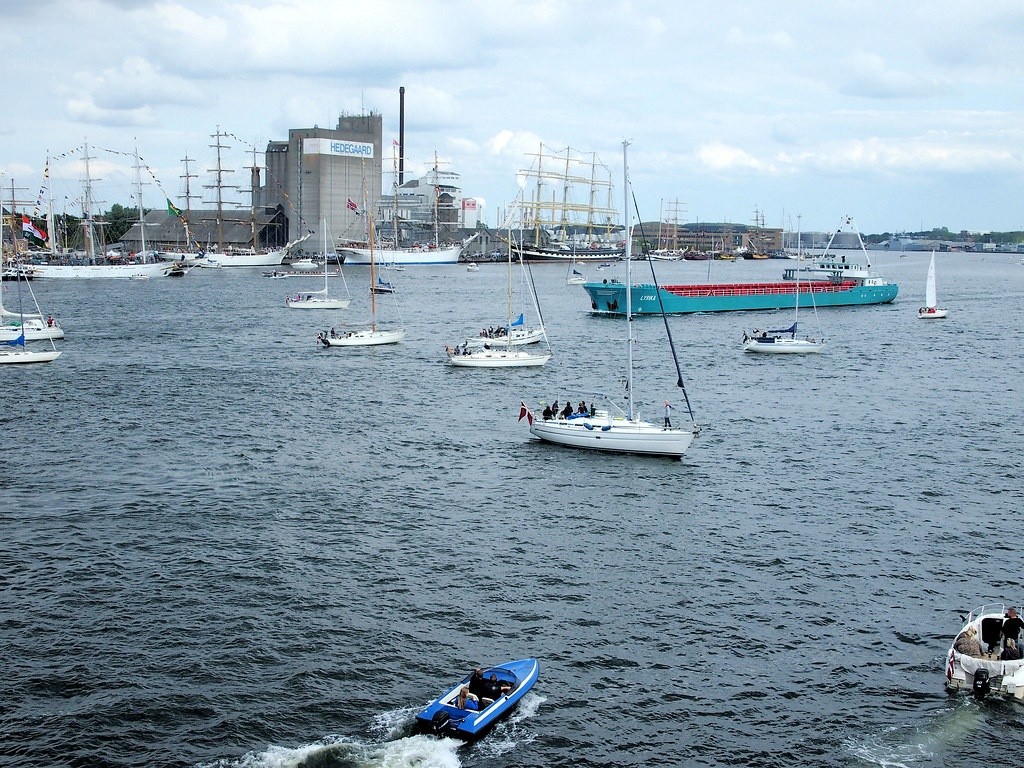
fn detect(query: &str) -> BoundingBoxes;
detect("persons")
[285,293,300,303]
[552,399,561,418]
[468,351,471,355]
[130,250,136,257]
[611,278,614,283]
[483,325,507,339]
[661,400,672,431]
[919,306,935,313]
[557,411,563,420]
[603,278,608,283]
[463,349,468,355]
[454,344,461,355]
[331,326,336,339]
[48,315,54,326]
[762,332,816,342]
[561,401,573,419]
[342,331,351,337]
[543,405,552,420]
[454,669,512,710]
[590,402,598,417]
[463,340,467,346]
[1000,608,1024,660]
[576,401,588,414]
[484,342,490,349]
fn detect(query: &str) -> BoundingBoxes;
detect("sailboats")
[284,217,354,311]
[370,229,396,295]
[739,212,830,355]
[0,222,64,366]
[315,205,407,348]
[446,222,554,369]
[334,145,481,268]
[565,228,589,286]
[917,248,949,320]
[530,142,703,461]
[2,118,342,282]
[459,203,548,349]
[383,180,406,272]
[899,229,909,260]
[495,143,839,272]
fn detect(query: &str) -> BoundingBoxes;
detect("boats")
[579,270,899,319]
[943,602,1024,706]
[0,289,65,344]
[416,658,540,739]
[466,259,480,273]
[781,213,884,284]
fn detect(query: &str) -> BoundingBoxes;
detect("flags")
[347,197,357,212]
[22,215,46,242]
